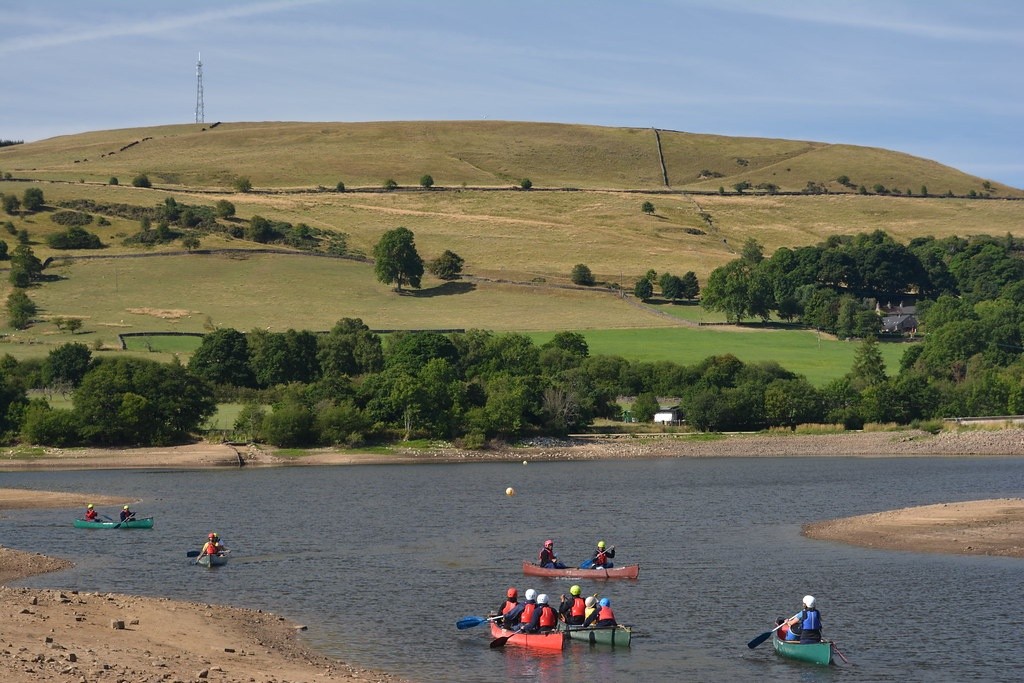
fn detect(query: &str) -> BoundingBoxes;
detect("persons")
[197,532,224,561]
[521,594,559,634]
[539,540,566,569]
[583,597,616,627]
[498,588,520,626]
[86,504,97,522]
[504,589,537,633]
[559,586,585,624]
[119,505,136,522]
[592,541,616,568]
[785,595,822,643]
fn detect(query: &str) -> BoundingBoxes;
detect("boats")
[553,617,632,647]
[771,632,835,667]
[488,611,566,651]
[521,558,640,580]
[72,517,155,528]
[197,548,232,567]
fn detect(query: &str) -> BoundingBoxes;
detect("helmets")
[88,503,93,508]
[803,595,816,607]
[585,596,596,607]
[597,540,606,547]
[124,505,129,509]
[599,597,610,607]
[214,532,217,537]
[545,539,553,548]
[537,593,548,604]
[209,533,214,537]
[507,588,517,598]
[570,584,580,595]
[525,588,537,602]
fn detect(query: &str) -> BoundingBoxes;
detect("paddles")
[113,511,136,529]
[566,596,571,601]
[456,614,504,630]
[93,510,116,520]
[580,545,615,568]
[186,552,232,558]
[490,624,530,650]
[747,609,805,650]
